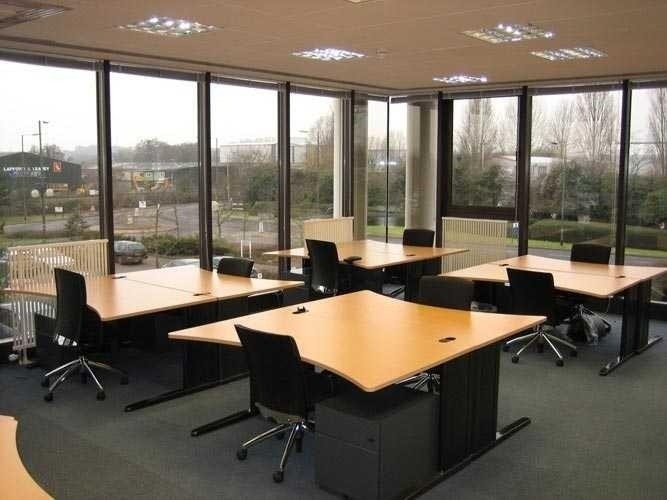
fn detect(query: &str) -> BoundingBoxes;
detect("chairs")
[235,324,314,482]
[306,238,352,296]
[391,275,474,394]
[503,267,577,366]
[218,258,254,277]
[404,228,434,247]
[247,291,284,314]
[558,243,612,342]
[42,268,129,402]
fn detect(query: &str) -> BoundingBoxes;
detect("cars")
[113,240,149,265]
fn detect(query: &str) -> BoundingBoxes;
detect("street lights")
[27,185,57,240]
[549,139,568,244]
[31,187,53,233]
[18,131,39,221]
[36,117,53,184]
[38,120,49,233]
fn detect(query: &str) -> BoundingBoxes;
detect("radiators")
[442,216,508,274]
[8,238,109,364]
[304,215,354,245]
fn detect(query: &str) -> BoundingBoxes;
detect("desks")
[437,263,641,376]
[336,239,470,296]
[287,289,549,478]
[365,237,366,243]
[1,415,53,500]
[168,306,474,500]
[262,244,424,300]
[114,265,304,385]
[454,266,457,267]
[489,254,667,355]
[13,276,218,413]
